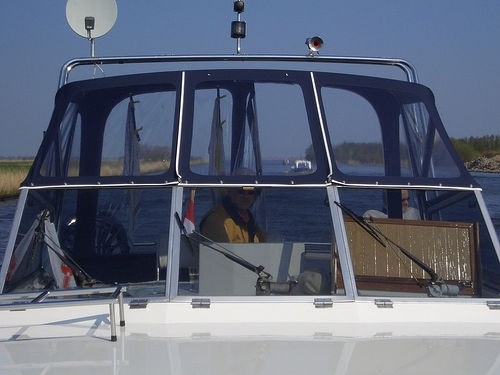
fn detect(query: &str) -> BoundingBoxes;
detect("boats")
[1,0,500,375]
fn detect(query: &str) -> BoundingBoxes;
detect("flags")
[207,98,226,208]
[120,103,143,213]
[181,188,198,238]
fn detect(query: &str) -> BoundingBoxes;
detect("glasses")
[238,189,256,194]
[402,198,409,201]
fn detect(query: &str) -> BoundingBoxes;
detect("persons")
[364,189,421,221]
[201,167,266,243]
